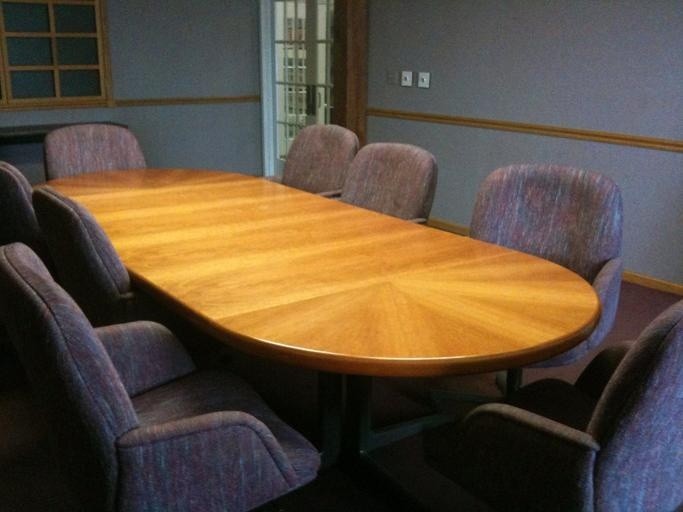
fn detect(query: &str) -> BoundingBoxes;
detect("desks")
[33,169,603,469]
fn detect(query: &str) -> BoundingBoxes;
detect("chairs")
[331,142,438,224]
[424,162,625,407]
[262,124,359,197]
[0,242,323,512]
[30,186,138,322]
[44,123,147,179]
[444,300,683,512]
[0,160,39,246]
